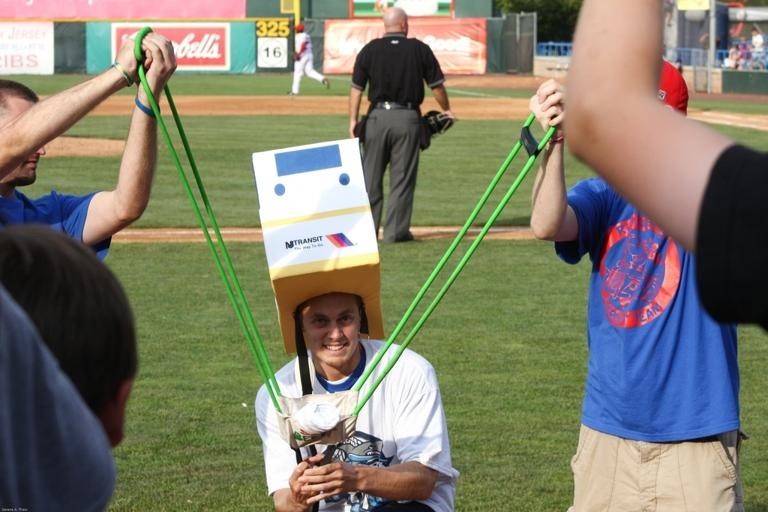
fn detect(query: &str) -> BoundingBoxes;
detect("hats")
[296,24,305,31]
[657,59,690,117]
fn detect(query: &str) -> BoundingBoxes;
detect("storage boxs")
[251,137,385,355]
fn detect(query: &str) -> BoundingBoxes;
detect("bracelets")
[112,61,134,88]
[134,95,162,117]
[546,137,564,143]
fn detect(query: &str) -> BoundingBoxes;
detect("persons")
[349,5,456,244]
[527,58,745,512]
[2,225,138,447]
[253,293,460,512]
[699,31,709,49]
[287,24,330,96]
[565,1,768,341]
[728,26,765,72]
[0,31,181,260]
[1,283,115,512]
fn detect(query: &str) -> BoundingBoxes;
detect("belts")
[374,101,410,110]
